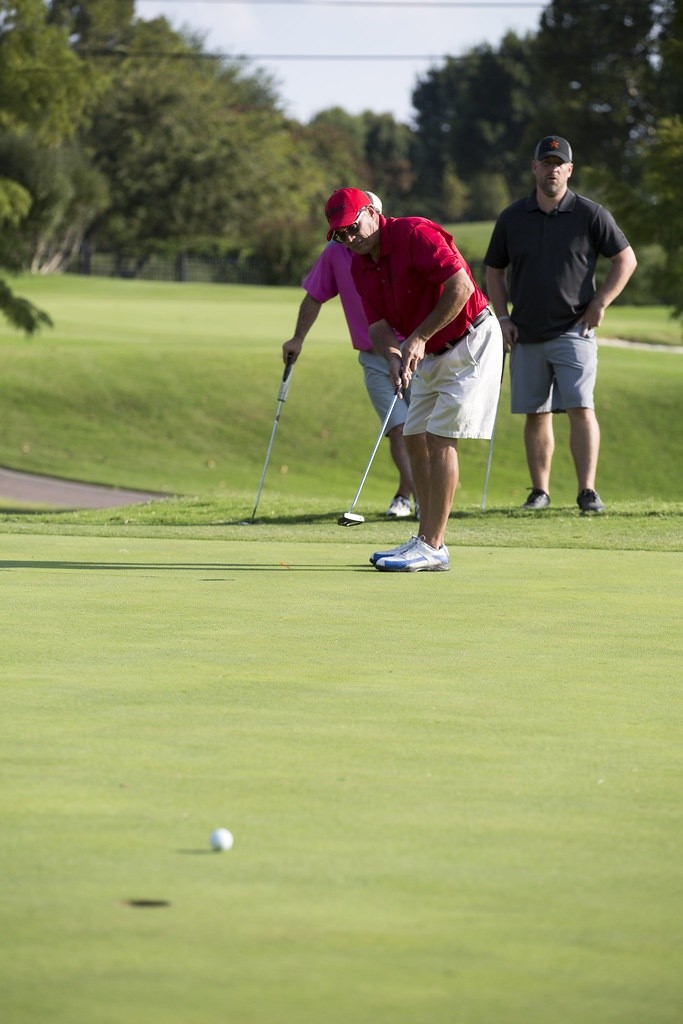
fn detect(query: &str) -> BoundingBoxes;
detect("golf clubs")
[338,361,404,527]
[480,344,506,509]
[237,352,298,526]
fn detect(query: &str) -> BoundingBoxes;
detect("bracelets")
[498,315,510,320]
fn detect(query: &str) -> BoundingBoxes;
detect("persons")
[281,191,422,521]
[325,188,504,573]
[483,137,638,515]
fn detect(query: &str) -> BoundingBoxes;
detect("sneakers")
[577,488,604,512]
[374,535,451,573]
[413,500,421,521]
[368,531,419,566]
[386,495,412,518]
[520,486,552,511]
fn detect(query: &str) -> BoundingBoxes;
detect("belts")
[427,307,490,358]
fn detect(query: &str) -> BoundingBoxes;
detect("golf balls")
[209,828,234,852]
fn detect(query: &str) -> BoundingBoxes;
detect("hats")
[324,187,372,242]
[534,136,573,163]
[363,190,383,214]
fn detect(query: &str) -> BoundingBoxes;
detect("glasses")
[332,211,363,243]
